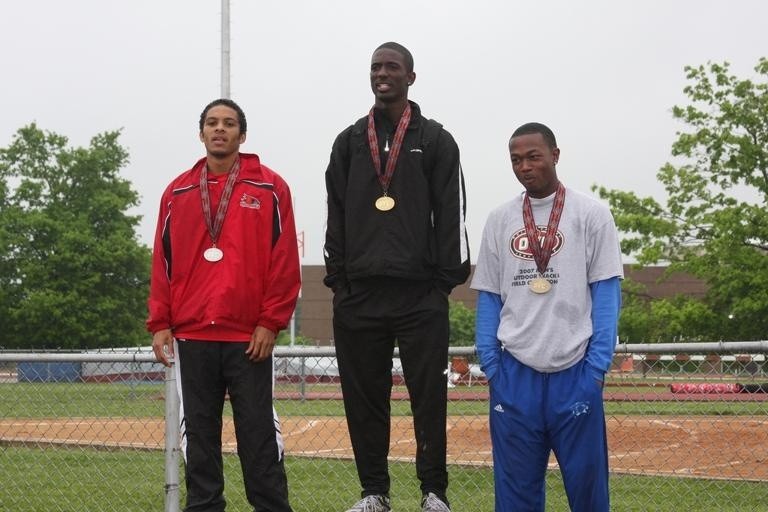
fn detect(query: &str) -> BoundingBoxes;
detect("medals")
[203,244,223,262]
[375,194,395,211]
[528,275,552,294]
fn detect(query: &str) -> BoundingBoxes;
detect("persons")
[146,100,301,512]
[469,122,626,512]
[322,42,471,512]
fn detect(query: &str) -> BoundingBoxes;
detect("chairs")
[448,354,473,387]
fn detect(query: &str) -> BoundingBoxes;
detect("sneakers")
[421,491,451,512]
[344,494,392,511]
